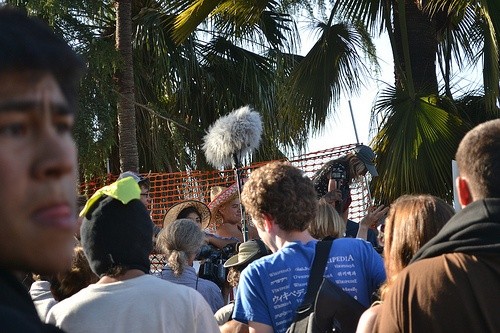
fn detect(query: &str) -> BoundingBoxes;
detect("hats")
[116,171,149,186]
[79,176,154,272]
[207,178,248,227]
[223,238,270,267]
[351,145,378,177]
[163,200,212,229]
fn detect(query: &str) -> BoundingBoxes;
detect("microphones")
[202,106,262,171]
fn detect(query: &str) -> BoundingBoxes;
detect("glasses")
[141,193,153,200]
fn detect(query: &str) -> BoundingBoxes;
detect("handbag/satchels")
[284,235,367,333]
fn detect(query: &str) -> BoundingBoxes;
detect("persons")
[1,11,81,333]
[26,120,500,333]
